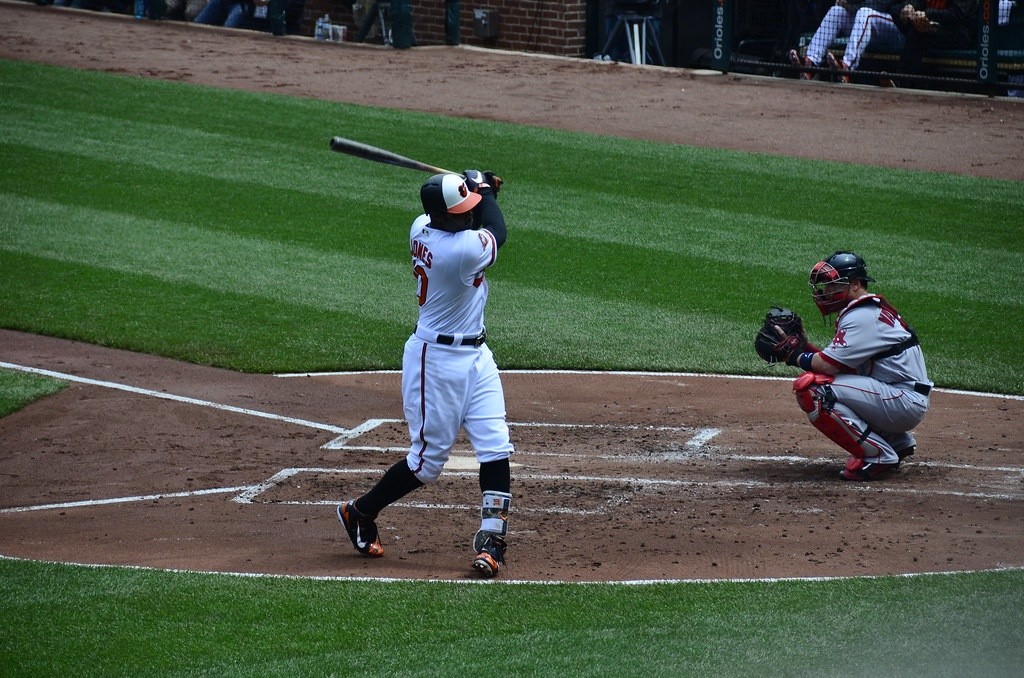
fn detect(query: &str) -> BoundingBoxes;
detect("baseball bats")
[329,135,468,183]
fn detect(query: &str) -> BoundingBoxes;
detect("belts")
[414,325,486,348]
[914,383,932,396]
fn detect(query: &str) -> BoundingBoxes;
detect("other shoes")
[790,49,816,80]
[827,52,852,84]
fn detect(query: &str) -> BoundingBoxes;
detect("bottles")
[134,0,144,18]
[315,14,331,40]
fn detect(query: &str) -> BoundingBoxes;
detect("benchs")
[799,33,1024,72]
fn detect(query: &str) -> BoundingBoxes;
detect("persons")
[53,0,305,35]
[337,169,512,574]
[757,252,932,479]
[789,0,931,82]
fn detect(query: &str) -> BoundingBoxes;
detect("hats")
[421,173,482,214]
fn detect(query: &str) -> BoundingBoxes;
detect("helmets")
[829,253,877,283]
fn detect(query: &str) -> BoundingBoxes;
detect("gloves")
[463,168,503,200]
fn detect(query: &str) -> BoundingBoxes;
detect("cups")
[329,25,347,42]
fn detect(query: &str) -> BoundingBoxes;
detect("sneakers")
[474,551,500,576]
[840,462,898,479]
[336,497,384,556]
[894,446,915,462]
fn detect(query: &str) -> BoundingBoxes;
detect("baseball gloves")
[755,308,807,364]
